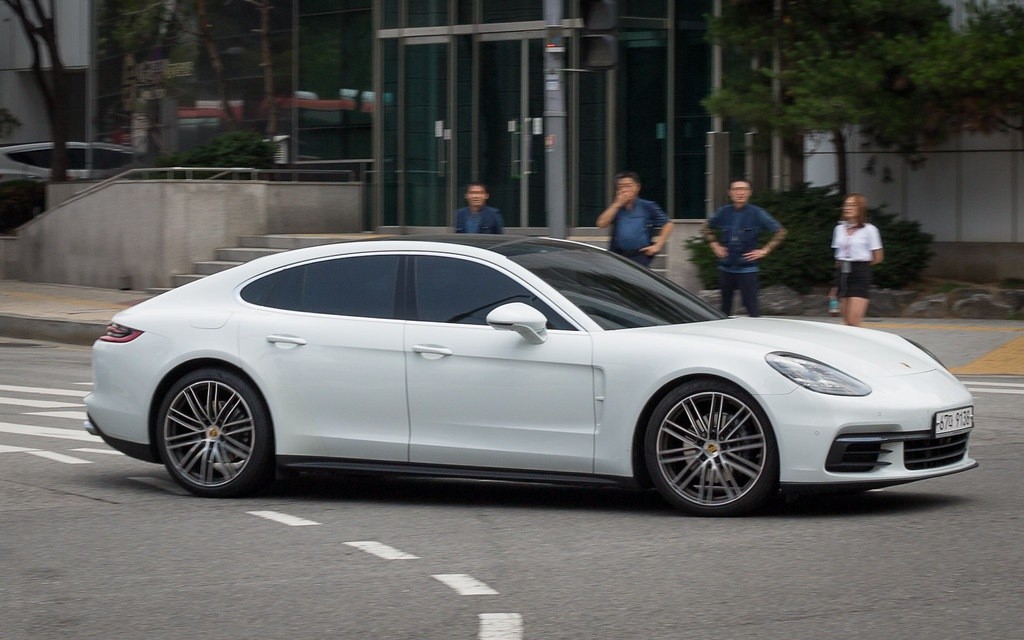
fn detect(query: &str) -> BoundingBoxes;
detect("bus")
[173,99,377,181]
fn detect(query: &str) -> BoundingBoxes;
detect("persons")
[700,177,788,317]
[596,172,675,267]
[830,193,884,327]
[452,183,505,297]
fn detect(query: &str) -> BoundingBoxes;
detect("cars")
[88,238,972,509]
[0,141,155,185]
